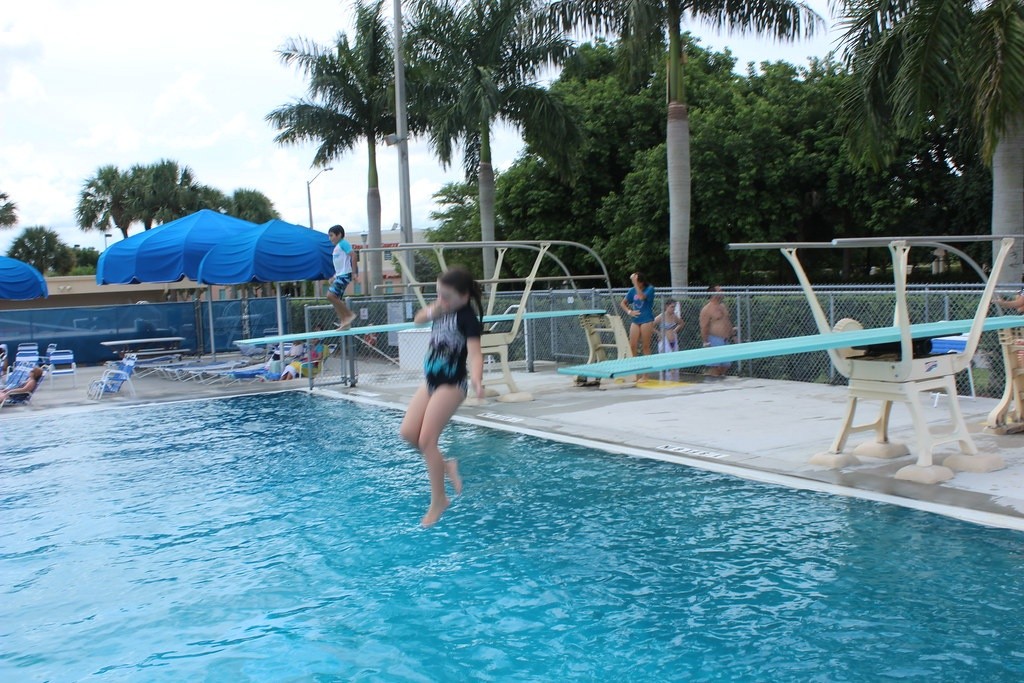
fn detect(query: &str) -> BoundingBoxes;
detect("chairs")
[928,319,975,408]
[0,328,338,411]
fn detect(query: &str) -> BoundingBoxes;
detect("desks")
[100,337,186,366]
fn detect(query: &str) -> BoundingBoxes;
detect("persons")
[280,338,323,381]
[0,348,42,402]
[400,269,484,526]
[620,272,655,383]
[327,225,361,331]
[990,289,1024,424]
[284,340,304,364]
[699,283,738,382]
[653,299,685,374]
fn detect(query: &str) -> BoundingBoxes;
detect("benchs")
[112,347,177,354]
[126,348,191,366]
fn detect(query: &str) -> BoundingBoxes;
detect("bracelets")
[734,334,737,337]
[427,308,432,320]
[628,310,632,314]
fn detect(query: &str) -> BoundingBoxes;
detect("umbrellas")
[0,255,48,301]
[196,219,352,335]
[97,209,260,361]
